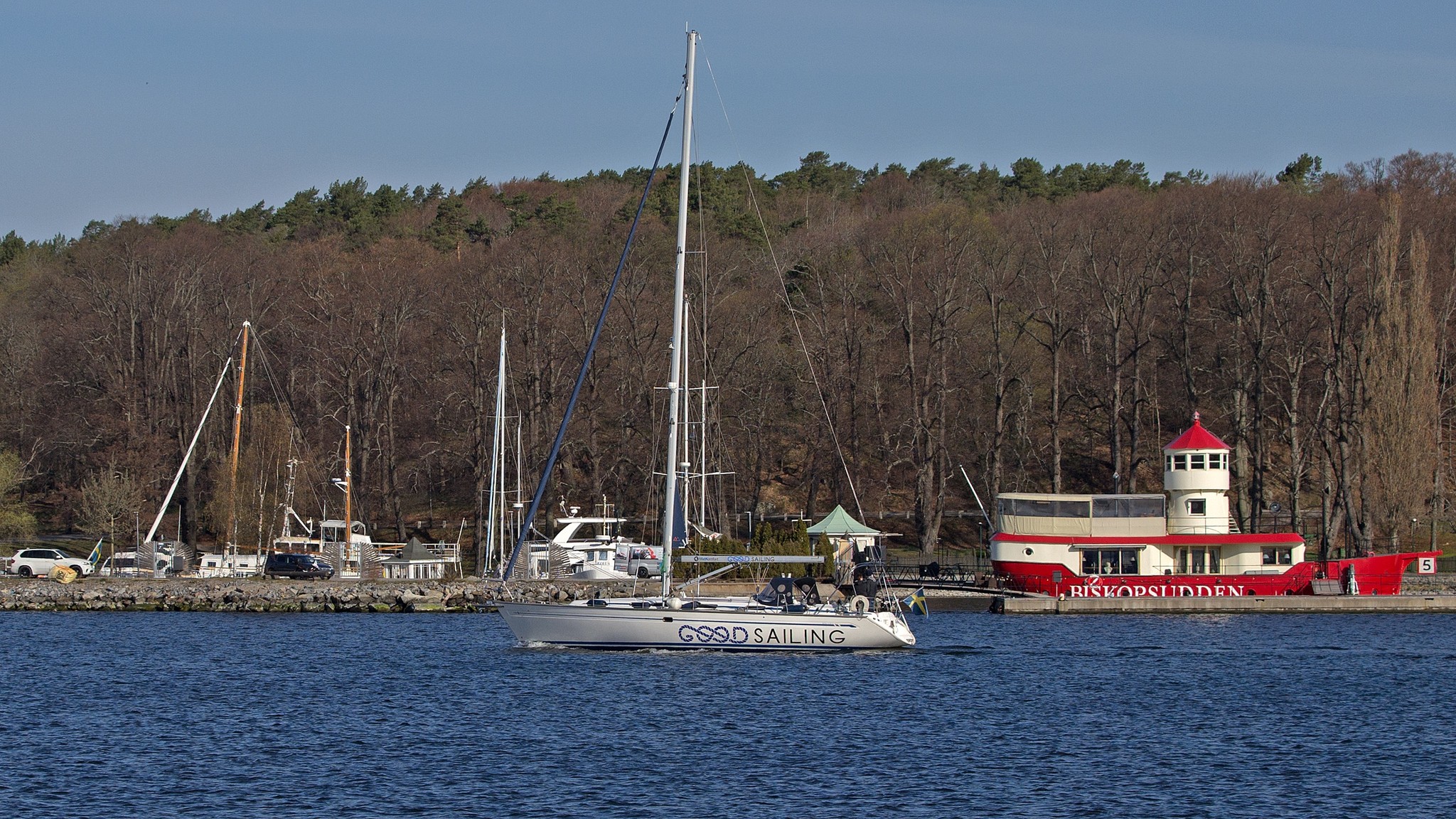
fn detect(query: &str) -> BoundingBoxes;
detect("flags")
[91,543,101,566]
[901,588,928,619]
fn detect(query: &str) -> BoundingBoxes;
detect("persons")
[874,594,884,613]
[854,573,877,612]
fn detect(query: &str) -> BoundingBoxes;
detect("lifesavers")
[1363,551,1369,557]
[1368,552,1375,557]
[851,594,870,611]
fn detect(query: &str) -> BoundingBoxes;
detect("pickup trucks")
[612,544,663,579]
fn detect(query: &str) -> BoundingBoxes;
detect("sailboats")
[471,21,918,653]
[100,318,385,579]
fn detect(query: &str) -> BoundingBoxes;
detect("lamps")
[1263,554,1269,558]
[1284,554,1290,558]
[1130,557,1135,561]
[1083,557,1086,561]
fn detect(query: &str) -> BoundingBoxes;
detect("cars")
[99,551,171,578]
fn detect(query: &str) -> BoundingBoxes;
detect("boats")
[496,492,663,579]
[958,409,1444,598]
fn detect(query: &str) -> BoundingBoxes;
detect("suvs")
[262,553,335,580]
[10,548,95,578]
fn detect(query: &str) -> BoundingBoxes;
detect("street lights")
[978,522,983,571]
[744,512,752,544]
[508,510,515,578]
[1410,518,1417,572]
[134,511,140,577]
